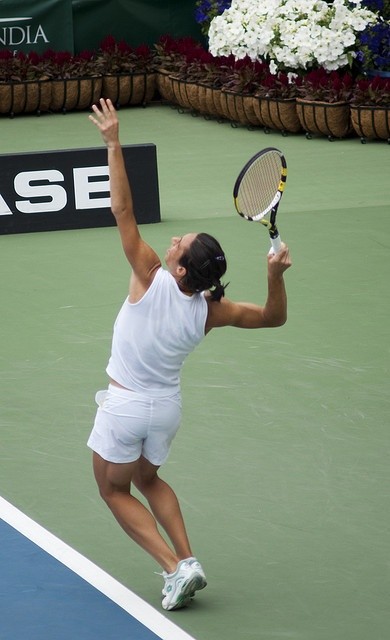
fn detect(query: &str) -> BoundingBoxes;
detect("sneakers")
[162,560,203,610]
[154,557,207,597]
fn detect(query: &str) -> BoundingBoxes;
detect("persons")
[86,97,291,611]
